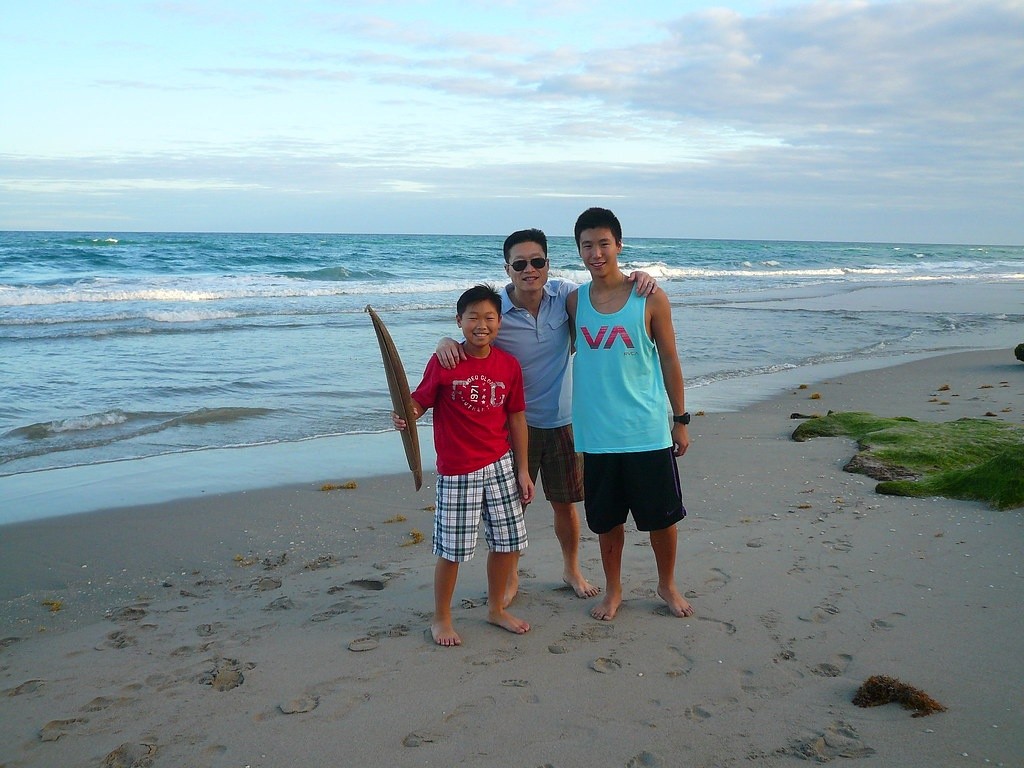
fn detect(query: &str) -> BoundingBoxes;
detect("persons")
[391,281,535,646]
[564,208,693,621]
[433,228,659,610]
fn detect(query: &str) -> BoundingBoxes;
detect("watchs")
[674,412,691,424]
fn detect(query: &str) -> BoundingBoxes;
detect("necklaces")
[591,273,624,304]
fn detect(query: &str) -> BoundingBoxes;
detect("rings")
[649,282,652,285]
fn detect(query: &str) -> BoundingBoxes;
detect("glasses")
[509,257,547,272]
